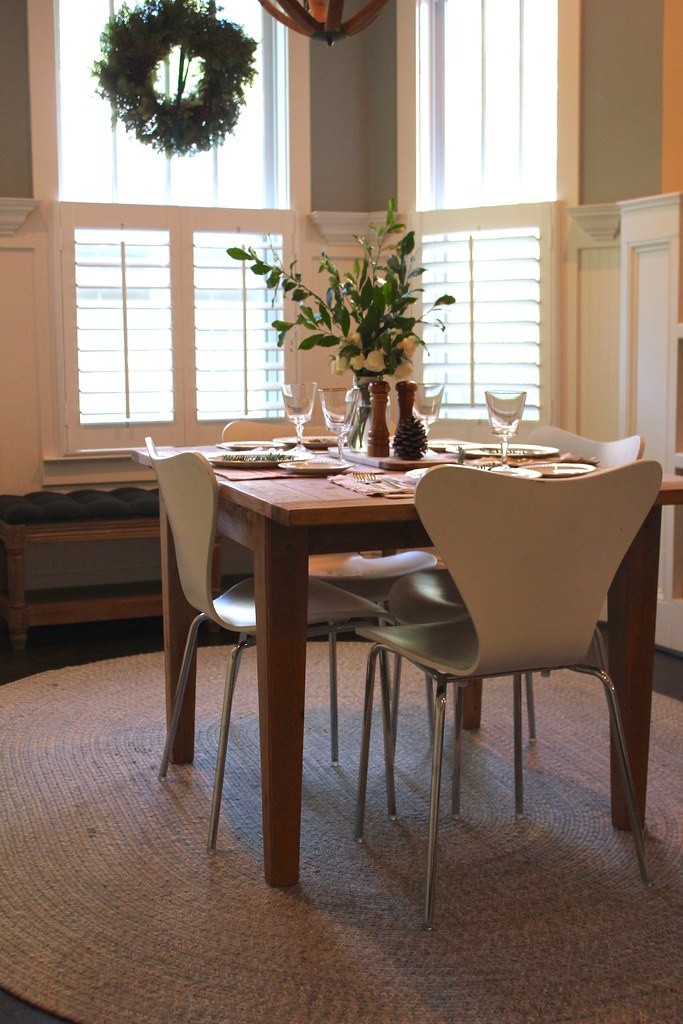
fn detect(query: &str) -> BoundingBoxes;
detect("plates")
[206,456,307,468]
[488,467,542,478]
[446,444,560,459]
[216,440,286,450]
[428,441,469,452]
[406,467,428,480]
[528,463,597,478]
[278,461,353,473]
[273,436,348,451]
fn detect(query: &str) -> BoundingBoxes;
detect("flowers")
[226,196,457,450]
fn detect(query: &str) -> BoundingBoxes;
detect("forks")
[363,472,383,492]
[352,470,374,490]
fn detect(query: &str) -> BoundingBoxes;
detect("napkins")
[326,473,420,496]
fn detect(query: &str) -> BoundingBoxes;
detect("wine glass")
[485,388,528,475]
[413,383,443,459]
[319,388,357,464]
[280,382,318,459]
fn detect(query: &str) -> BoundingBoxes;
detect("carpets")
[0,640,683,1024]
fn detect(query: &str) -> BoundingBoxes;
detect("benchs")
[0,488,222,654]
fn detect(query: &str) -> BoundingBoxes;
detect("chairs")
[144,419,652,925]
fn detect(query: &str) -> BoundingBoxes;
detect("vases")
[350,376,393,451]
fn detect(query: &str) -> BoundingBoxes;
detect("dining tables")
[131,440,682,889]
